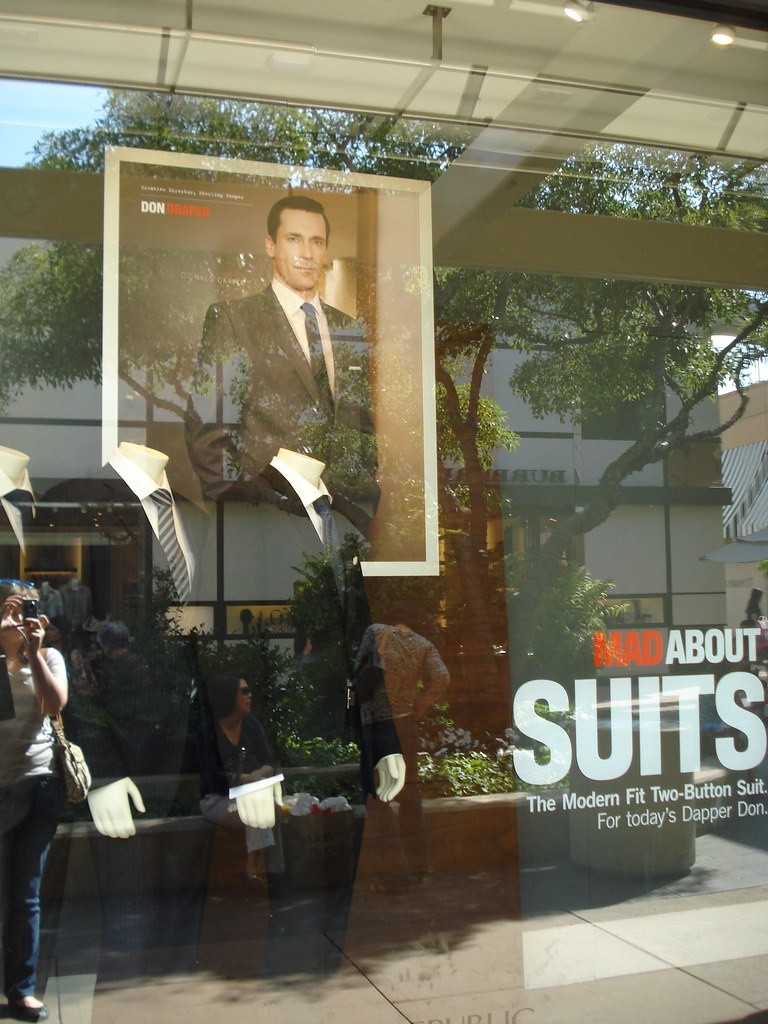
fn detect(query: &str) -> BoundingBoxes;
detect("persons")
[198,672,286,910]
[78,622,146,776]
[0,579,69,1022]
[201,449,406,971]
[0,442,211,979]
[184,195,380,561]
[356,603,451,888]
[745,588,763,666]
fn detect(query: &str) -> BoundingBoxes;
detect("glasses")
[238,686,251,695]
[0,579,34,590]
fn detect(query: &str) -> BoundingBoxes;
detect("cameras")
[23,599,38,621]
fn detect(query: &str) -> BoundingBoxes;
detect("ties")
[4,489,34,554]
[149,488,190,607]
[301,303,334,422]
[313,495,345,610]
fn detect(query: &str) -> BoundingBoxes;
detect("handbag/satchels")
[39,648,93,803]
[354,625,393,705]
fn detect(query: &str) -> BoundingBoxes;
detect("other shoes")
[241,870,267,887]
[363,875,402,894]
[8,1000,49,1022]
